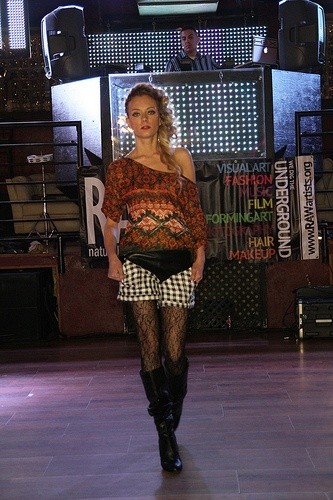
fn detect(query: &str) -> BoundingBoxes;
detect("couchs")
[314,159,333,233]
[6,173,80,233]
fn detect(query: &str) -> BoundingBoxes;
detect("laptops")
[234,36,278,69]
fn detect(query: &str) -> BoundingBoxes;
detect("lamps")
[136,0,220,16]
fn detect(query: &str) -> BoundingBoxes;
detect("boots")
[140,366,182,473]
[163,357,190,428]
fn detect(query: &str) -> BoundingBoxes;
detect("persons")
[100,81,209,472]
[165,27,219,71]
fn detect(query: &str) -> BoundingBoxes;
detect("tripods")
[25,162,61,254]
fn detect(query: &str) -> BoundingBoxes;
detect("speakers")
[278,0,326,69]
[297,285,333,338]
[41,5,90,83]
